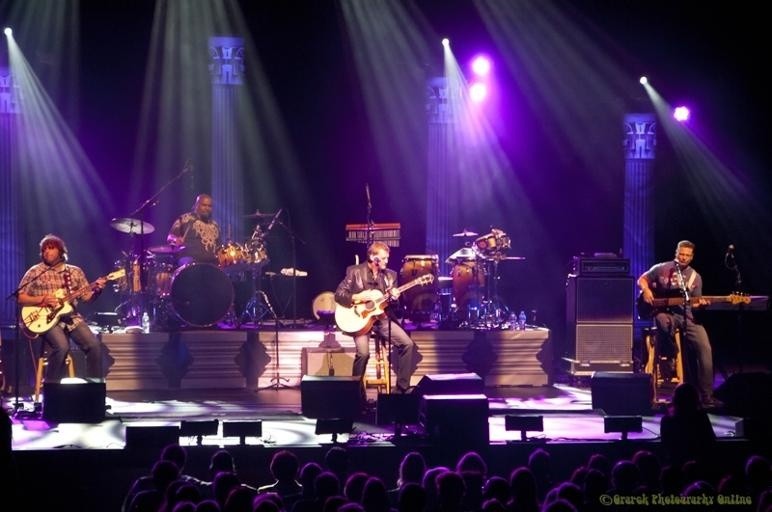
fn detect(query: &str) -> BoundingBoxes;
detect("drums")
[313,291,337,319]
[114,263,152,295]
[453,257,486,319]
[400,255,440,322]
[218,240,248,271]
[495,233,510,250]
[475,234,497,255]
[162,263,234,327]
[147,272,170,296]
[244,240,270,269]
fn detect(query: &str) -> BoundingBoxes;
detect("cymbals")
[109,218,155,234]
[453,232,478,237]
[148,245,185,254]
[243,214,275,217]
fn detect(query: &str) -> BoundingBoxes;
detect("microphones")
[674,258,679,267]
[370,255,382,263]
[62,252,67,262]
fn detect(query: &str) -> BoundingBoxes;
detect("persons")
[637,240,725,408]
[124,446,772,511]
[335,241,413,394]
[167,193,222,265]
[19,235,112,409]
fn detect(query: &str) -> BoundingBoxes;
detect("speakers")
[592,371,653,416]
[410,373,484,394]
[302,375,365,419]
[566,277,635,360]
[43,383,105,422]
[418,396,489,440]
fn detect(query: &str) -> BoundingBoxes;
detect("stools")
[33,344,77,401]
[644,328,685,398]
[362,335,392,396]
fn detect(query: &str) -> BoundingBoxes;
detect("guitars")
[18,269,125,339]
[638,288,751,319]
[335,274,435,336]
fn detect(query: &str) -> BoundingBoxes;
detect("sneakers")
[702,394,725,409]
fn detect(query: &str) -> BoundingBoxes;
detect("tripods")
[464,264,511,329]
[236,265,283,329]
[254,288,299,392]
